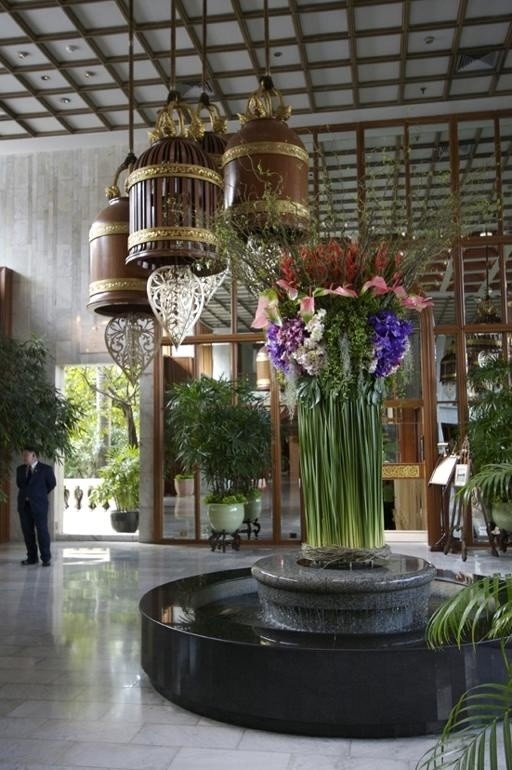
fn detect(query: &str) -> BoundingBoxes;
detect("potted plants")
[173,473,196,496]
[458,351,511,533]
[238,393,271,523]
[88,441,140,533]
[163,373,267,534]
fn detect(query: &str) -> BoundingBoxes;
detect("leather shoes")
[21,556,38,565]
[42,560,50,566]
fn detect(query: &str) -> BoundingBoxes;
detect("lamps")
[438,226,504,406]
[86,1,310,389]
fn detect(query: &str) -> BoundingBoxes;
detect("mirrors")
[152,331,281,547]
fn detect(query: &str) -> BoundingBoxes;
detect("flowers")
[160,118,510,550]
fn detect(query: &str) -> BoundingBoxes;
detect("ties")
[27,466,31,481]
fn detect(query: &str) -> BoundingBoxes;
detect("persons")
[16,446,56,567]
